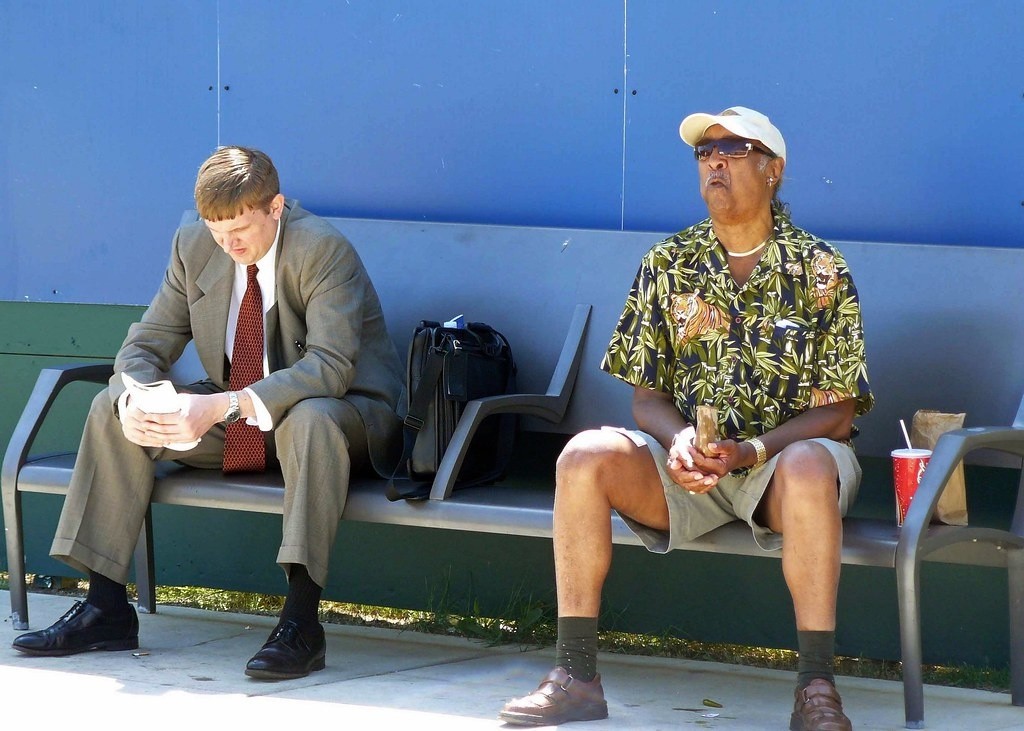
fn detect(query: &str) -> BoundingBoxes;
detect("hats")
[679,107,786,167]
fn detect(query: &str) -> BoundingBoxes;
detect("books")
[449,314,465,343]
[121,372,201,451]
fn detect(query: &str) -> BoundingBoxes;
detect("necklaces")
[724,234,769,259]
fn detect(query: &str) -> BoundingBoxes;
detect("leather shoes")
[496,666,608,725]
[245,617,326,678]
[790,679,852,731]
[11,600,139,655]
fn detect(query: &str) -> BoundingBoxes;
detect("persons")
[501,105,875,731]
[12,145,407,678]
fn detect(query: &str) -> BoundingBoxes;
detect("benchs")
[0,214,1024,731]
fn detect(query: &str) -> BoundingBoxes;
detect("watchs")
[225,391,240,423]
[728,438,766,478]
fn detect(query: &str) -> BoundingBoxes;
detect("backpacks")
[384,320,518,502]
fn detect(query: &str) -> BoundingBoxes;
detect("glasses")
[694,138,773,161]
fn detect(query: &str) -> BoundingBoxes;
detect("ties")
[222,262,266,478]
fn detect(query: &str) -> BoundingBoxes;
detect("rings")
[689,491,696,496]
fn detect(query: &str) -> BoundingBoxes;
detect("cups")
[890,449,933,536]
